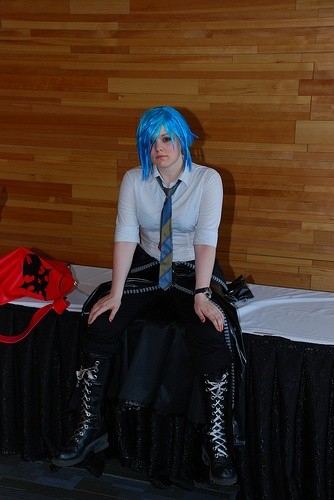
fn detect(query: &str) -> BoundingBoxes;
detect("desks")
[0,265,334,500]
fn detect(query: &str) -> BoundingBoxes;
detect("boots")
[50,352,116,468]
[198,369,240,487]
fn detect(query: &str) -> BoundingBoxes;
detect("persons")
[51,105,247,484]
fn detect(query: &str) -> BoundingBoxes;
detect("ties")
[155,175,184,293]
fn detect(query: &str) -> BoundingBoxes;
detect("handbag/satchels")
[0,246,78,345]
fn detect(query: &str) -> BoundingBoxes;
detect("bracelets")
[194,287,212,298]
